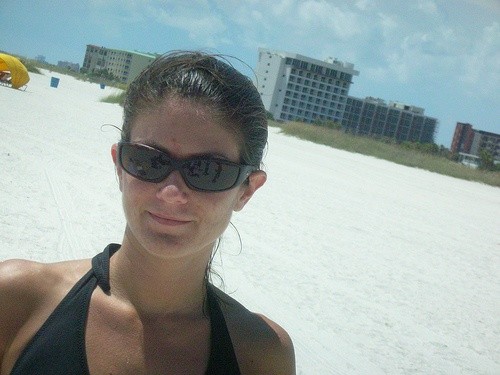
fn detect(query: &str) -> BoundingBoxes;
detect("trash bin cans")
[100,83,105,90]
[50,77,59,88]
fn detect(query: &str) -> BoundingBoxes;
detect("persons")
[0,47,296,375]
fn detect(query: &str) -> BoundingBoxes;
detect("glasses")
[116,141,257,193]
